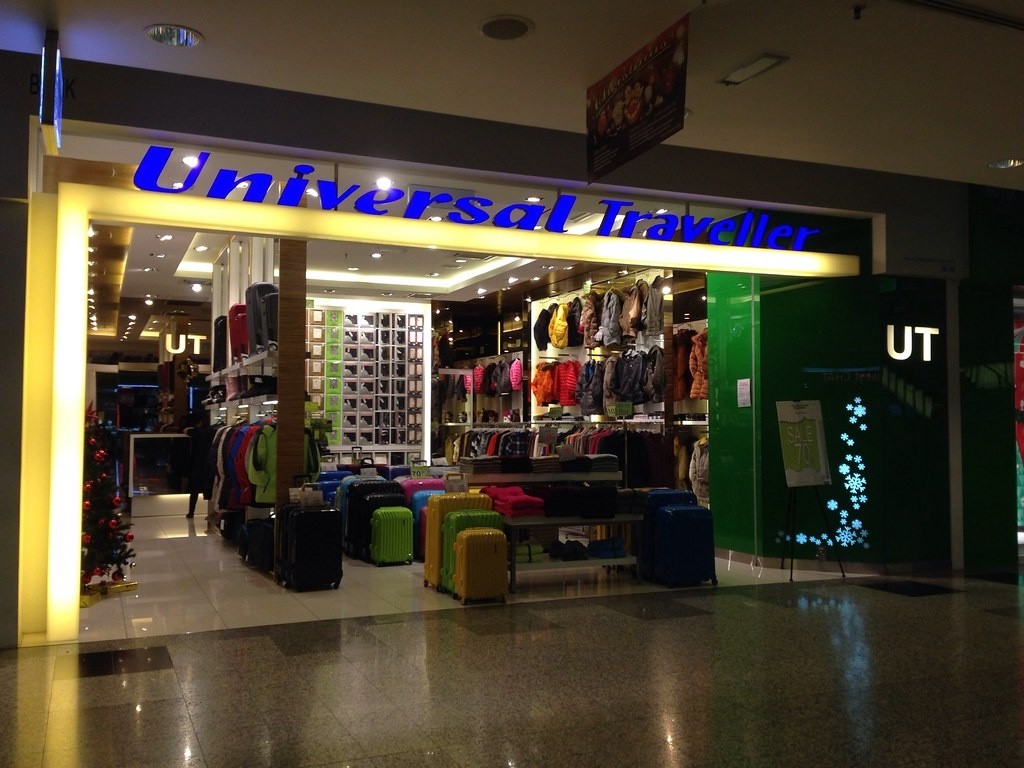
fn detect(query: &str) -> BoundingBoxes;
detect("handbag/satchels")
[240,513,274,562]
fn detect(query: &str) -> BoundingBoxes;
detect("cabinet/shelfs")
[205,347,277,410]
[305,300,424,470]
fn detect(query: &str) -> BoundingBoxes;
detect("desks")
[504,515,643,594]
[466,471,623,575]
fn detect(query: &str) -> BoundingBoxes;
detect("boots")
[230,375,277,400]
[201,385,226,405]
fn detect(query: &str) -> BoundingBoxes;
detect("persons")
[183,416,216,518]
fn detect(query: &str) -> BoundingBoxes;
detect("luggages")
[424,473,507,604]
[275,483,343,592]
[309,455,447,567]
[229,305,248,365]
[637,488,718,588]
[213,315,227,373]
[246,284,278,358]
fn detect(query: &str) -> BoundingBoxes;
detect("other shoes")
[187,514,193,518]
